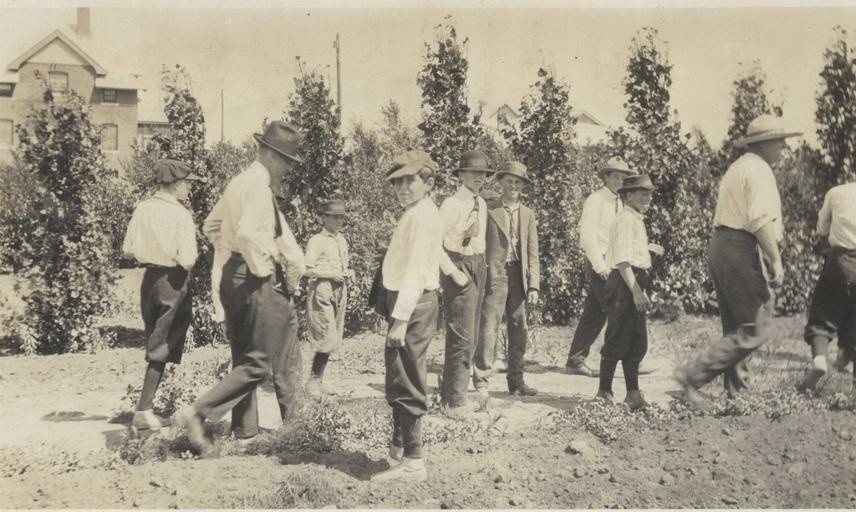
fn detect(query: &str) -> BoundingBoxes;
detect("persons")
[213,180,304,438]
[302,197,355,396]
[170,121,303,454]
[473,162,540,396]
[368,149,440,483]
[595,174,651,413]
[675,115,805,402]
[438,151,498,417]
[797,156,854,395]
[123,160,208,430]
[565,155,658,376]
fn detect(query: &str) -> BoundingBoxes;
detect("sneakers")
[133,409,172,432]
[176,403,218,461]
[673,366,706,404]
[566,360,601,378]
[369,453,428,486]
[594,392,618,407]
[236,433,263,449]
[622,389,653,411]
[440,386,493,413]
[507,383,539,397]
[802,355,827,393]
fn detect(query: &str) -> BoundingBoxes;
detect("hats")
[496,160,532,186]
[597,155,637,179]
[383,149,436,186]
[152,159,200,185]
[732,113,804,148]
[251,119,307,165]
[616,173,658,193]
[451,151,496,178]
[317,199,346,217]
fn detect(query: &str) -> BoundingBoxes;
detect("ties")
[509,210,519,263]
[462,195,480,248]
[273,193,284,241]
